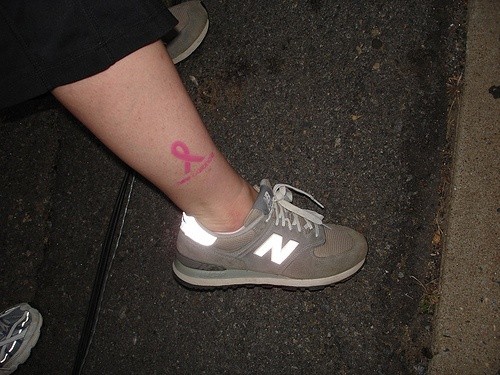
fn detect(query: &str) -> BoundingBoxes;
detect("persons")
[0,0,369,291]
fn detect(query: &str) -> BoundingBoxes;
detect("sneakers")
[171,180,369,293]
[0,302,44,375]
[164,1,209,66]
